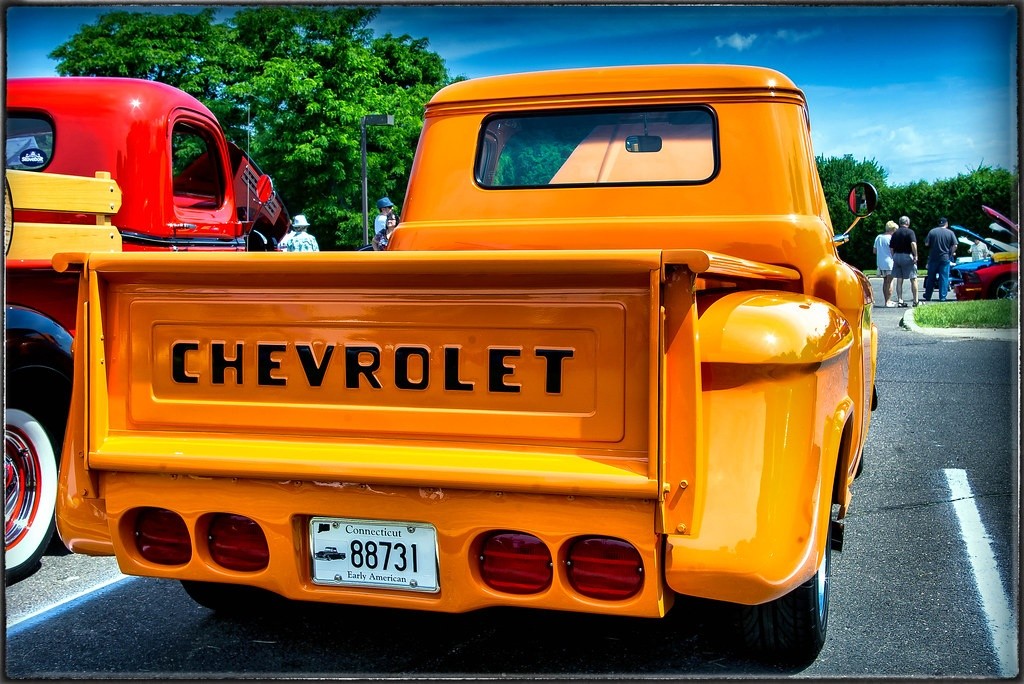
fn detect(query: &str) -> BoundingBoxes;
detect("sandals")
[912,301,925,307]
[899,302,908,307]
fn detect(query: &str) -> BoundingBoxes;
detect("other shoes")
[885,300,896,307]
[922,293,930,300]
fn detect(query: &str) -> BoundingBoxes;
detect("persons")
[888,216,921,308]
[371,213,401,251]
[872,220,897,307]
[374,196,395,233]
[968,238,988,262]
[920,217,959,303]
[280,214,320,253]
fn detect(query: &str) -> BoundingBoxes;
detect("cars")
[925,205,1019,303]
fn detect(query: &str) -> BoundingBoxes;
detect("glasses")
[387,217,396,221]
[387,205,392,210]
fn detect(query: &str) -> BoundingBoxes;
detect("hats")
[937,217,948,226]
[291,215,310,228]
[377,198,394,208]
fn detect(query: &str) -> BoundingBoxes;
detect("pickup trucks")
[1,78,278,582]
[52,63,878,667]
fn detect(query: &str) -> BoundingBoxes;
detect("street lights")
[360,114,395,245]
